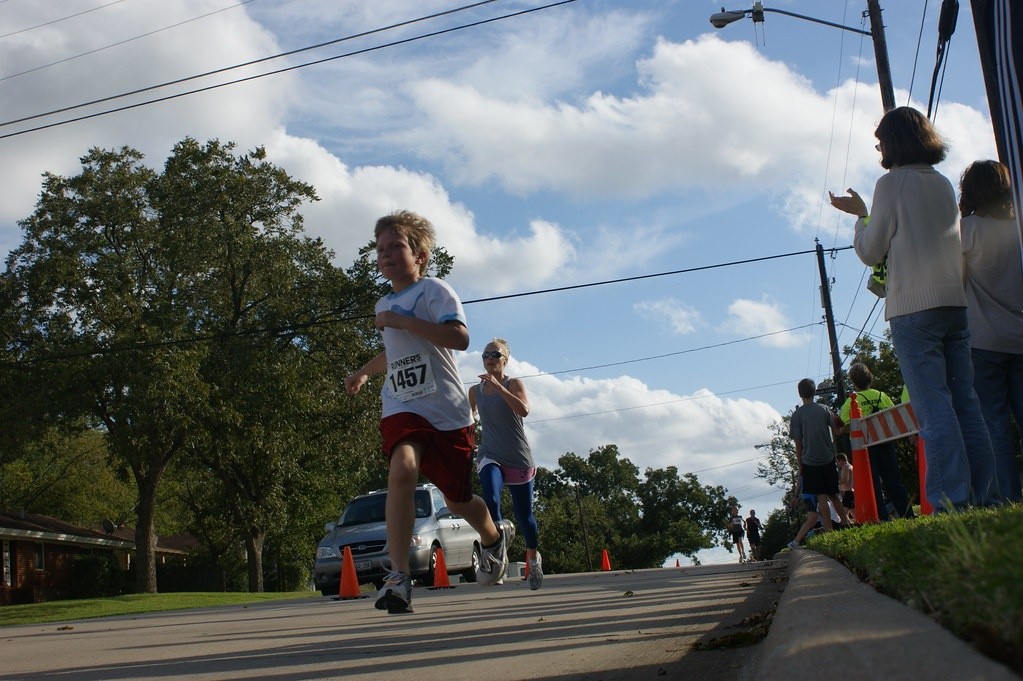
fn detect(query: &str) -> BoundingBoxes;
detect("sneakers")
[475,519,516,587]
[787,541,799,551]
[374,556,414,615]
[528,551,544,591]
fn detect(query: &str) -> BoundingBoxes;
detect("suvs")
[313,483,483,597]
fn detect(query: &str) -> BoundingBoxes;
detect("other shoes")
[521,576,527,580]
[497,574,508,585]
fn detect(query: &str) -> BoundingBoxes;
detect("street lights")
[754,442,805,547]
[708,4,896,116]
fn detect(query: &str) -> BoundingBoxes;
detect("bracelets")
[859,216,866,218]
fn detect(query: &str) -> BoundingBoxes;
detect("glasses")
[481,351,507,359]
[875,143,882,151]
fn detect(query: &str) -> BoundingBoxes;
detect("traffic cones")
[331,545,371,601]
[675,560,681,568]
[917,427,932,517]
[600,549,612,571]
[520,549,529,581]
[426,548,457,590]
[849,390,882,528]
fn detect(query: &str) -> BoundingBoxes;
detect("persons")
[469,338,543,590]
[787,362,911,551]
[344,211,517,614]
[958,160,1023,503]
[743,510,763,561]
[829,106,1003,519]
[728,506,746,565]
